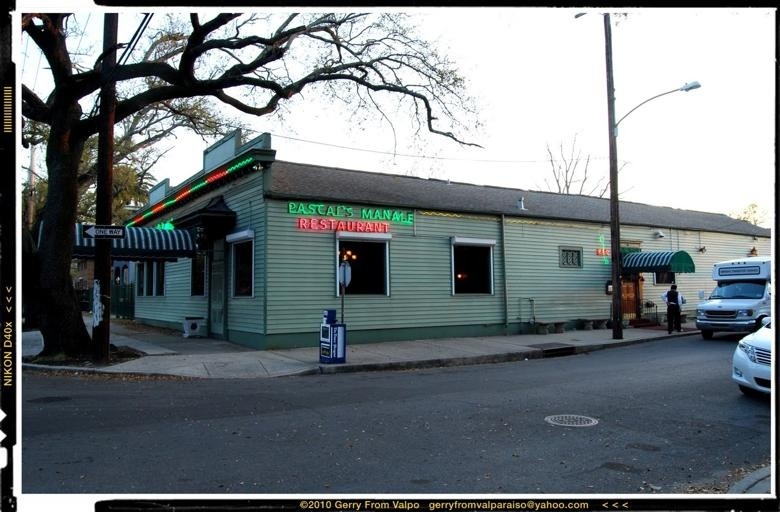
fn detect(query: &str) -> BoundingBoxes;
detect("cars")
[732,319,771,395]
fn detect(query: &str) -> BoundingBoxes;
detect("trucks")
[696,255,771,340]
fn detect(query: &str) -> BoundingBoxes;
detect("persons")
[660,283,687,333]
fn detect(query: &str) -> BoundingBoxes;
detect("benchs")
[529,316,610,334]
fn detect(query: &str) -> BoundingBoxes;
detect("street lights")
[574,12,702,340]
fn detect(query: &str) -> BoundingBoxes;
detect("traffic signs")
[83,225,125,239]
